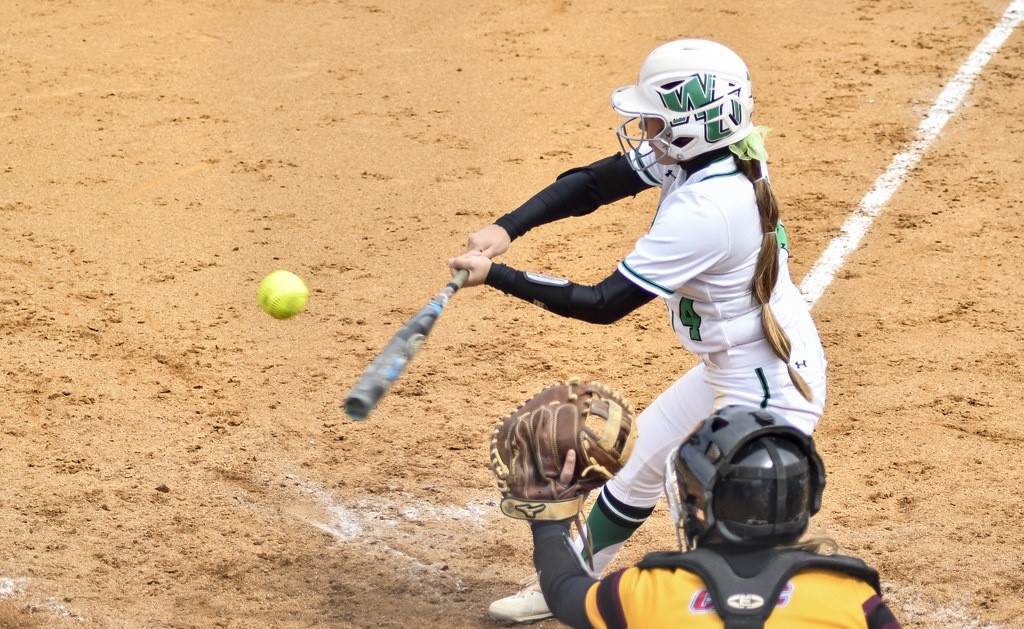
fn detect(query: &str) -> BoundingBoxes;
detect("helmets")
[610,39,755,172]
[662,404,827,553]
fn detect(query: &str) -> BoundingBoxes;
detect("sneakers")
[488,575,555,627]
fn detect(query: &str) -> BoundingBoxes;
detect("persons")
[493,405,916,629]
[444,40,831,625]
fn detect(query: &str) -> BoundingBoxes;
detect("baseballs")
[256,269,307,320]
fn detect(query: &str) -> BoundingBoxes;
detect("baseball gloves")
[489,377,639,523]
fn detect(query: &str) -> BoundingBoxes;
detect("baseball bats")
[344,265,468,421]
